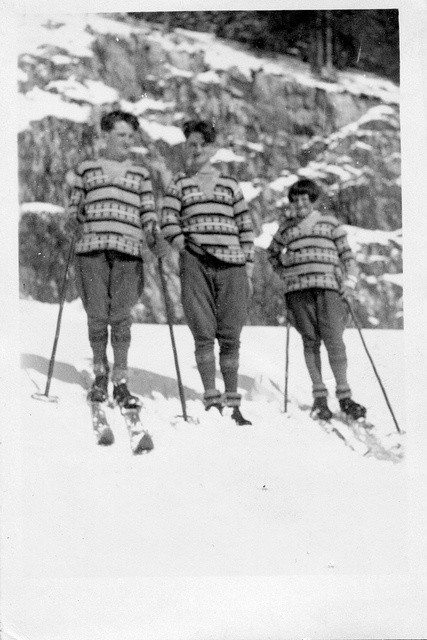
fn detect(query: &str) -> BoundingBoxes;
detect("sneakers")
[90,376,108,402]
[339,397,366,421]
[113,384,139,408]
[228,406,252,425]
[205,403,223,416]
[311,397,333,420]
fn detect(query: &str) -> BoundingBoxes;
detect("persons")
[60,110,160,408]
[265,179,366,418]
[160,120,254,424]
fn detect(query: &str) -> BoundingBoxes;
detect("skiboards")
[88,388,154,455]
[301,402,403,461]
